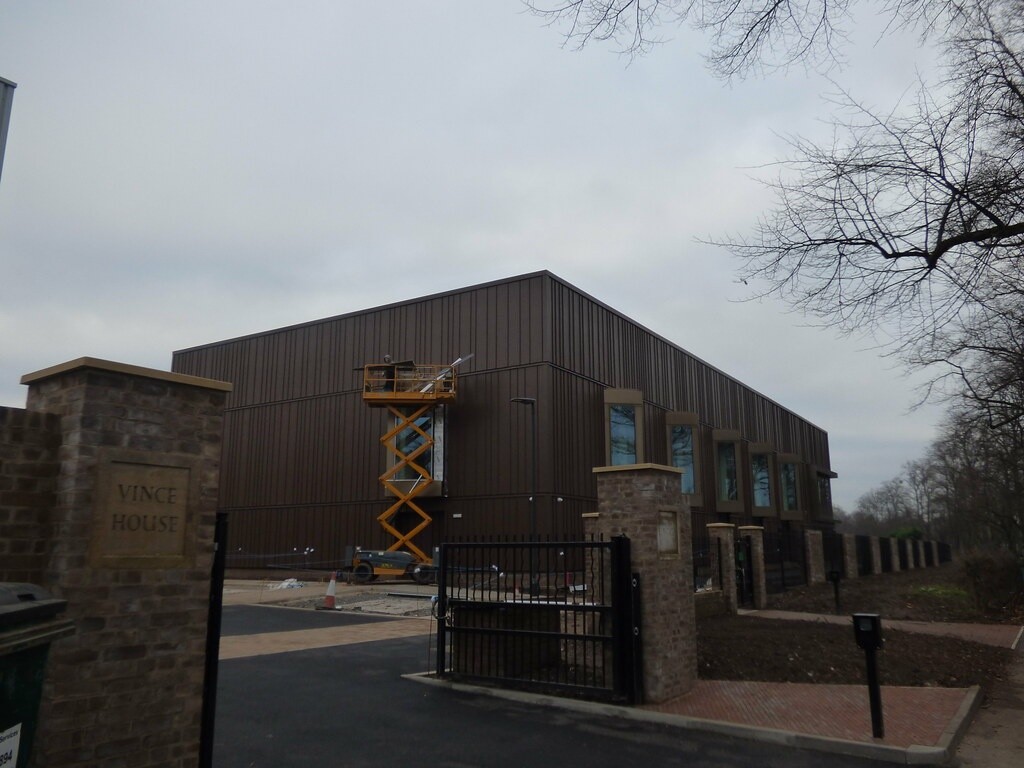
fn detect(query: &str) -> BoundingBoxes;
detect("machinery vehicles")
[351,362,457,583]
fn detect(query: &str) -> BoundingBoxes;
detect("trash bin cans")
[0,580,78,768]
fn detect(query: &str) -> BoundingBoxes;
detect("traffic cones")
[325,572,338,609]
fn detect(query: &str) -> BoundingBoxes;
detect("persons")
[383,355,394,389]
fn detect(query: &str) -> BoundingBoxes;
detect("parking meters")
[850,611,888,740]
